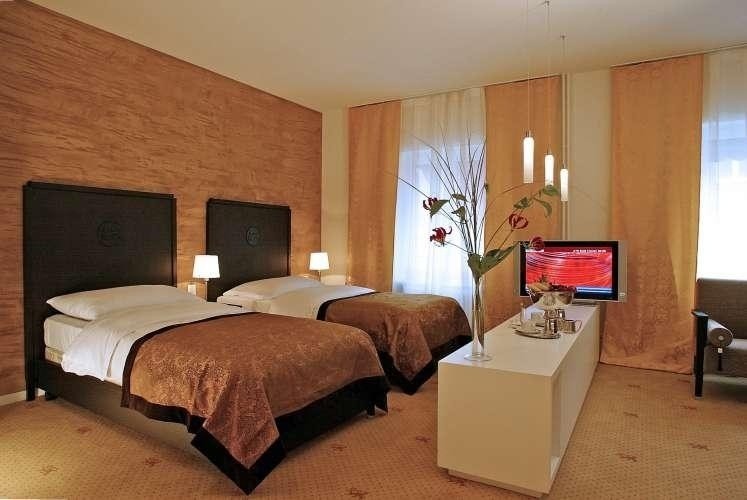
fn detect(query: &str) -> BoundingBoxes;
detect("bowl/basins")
[528,290,575,309]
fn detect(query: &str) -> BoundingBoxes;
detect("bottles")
[188,280,196,296]
[520,300,526,321]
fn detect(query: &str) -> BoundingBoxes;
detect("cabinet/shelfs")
[436,299,602,499]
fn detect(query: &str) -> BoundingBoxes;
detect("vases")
[463,278,491,361]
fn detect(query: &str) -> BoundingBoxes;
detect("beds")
[21,181,393,496]
[205,198,474,396]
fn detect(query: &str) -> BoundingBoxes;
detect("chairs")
[690,278,747,398]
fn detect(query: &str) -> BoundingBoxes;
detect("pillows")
[46,284,206,321]
[223,276,322,303]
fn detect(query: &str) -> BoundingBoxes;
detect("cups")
[523,309,583,334]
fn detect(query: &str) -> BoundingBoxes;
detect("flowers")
[384,127,557,347]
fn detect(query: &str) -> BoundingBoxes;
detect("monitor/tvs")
[512,241,627,303]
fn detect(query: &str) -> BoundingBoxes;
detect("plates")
[517,329,540,333]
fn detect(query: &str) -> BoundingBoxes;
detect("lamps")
[524,1,536,183]
[193,254,221,302]
[308,252,330,283]
[559,34,569,203]
[543,0,556,189]
